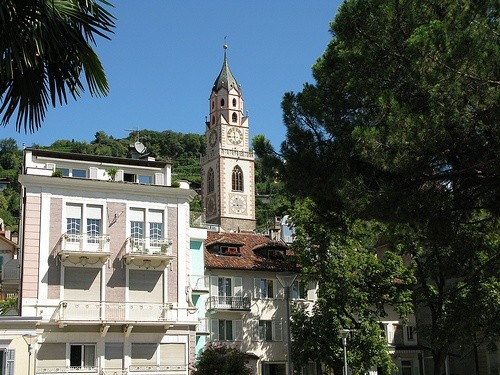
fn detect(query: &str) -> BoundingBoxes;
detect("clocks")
[209,129,218,147]
[227,127,243,145]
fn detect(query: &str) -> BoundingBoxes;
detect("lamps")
[13,246,18,260]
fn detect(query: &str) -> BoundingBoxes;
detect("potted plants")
[160,243,173,255]
[132,243,142,254]
[224,249,238,255]
[105,167,118,181]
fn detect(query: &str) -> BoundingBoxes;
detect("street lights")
[274,270,302,375]
[340,328,351,375]
